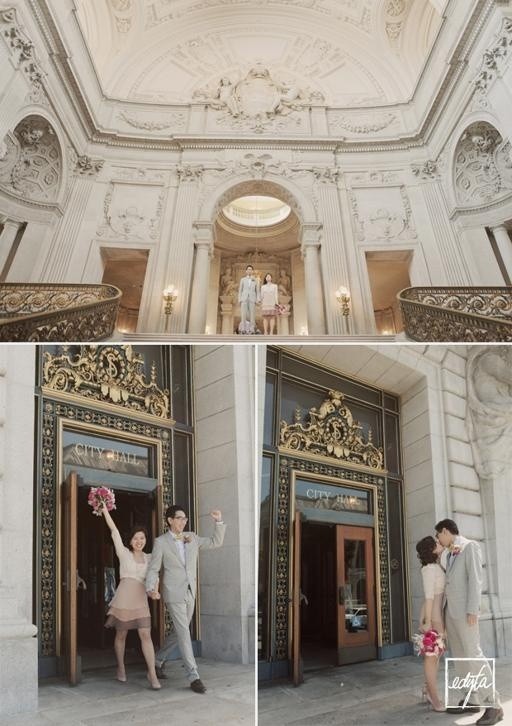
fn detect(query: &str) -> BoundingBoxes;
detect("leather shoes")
[190,678,207,694]
[115,669,127,682]
[476,706,504,725]
[155,667,169,680]
[447,699,481,713]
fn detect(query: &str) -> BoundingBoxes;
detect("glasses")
[174,517,189,521]
[435,533,440,539]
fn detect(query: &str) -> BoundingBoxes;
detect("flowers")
[86,486,119,516]
[408,628,448,658]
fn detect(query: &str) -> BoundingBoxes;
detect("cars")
[344,607,368,628]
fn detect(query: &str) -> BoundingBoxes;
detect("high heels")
[146,671,162,690]
[422,686,447,712]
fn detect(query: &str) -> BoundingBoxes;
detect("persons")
[238,265,279,336]
[98,498,225,695]
[416,518,502,726]
